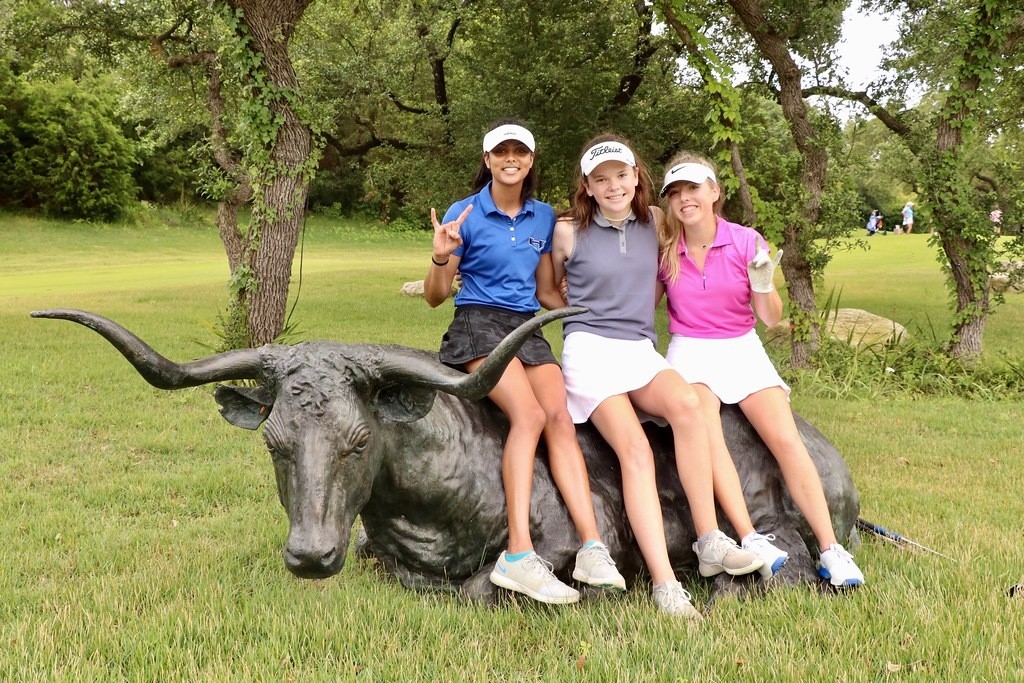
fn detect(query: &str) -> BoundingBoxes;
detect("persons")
[901,202,915,234]
[867,209,886,236]
[990,206,1002,237]
[424,119,865,617]
[432,256,449,266]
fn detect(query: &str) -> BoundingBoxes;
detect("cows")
[28,306,862,612]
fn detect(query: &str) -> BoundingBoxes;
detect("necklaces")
[605,209,632,221]
[696,232,715,248]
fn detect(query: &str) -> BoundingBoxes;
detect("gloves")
[747,235,785,293]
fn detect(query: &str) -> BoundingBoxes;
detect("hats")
[580,141,636,176]
[907,202,914,205]
[482,124,534,154]
[660,163,717,199]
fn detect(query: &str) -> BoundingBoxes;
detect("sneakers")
[816,543,865,585]
[692,530,764,577]
[573,542,626,590]
[489,550,580,604]
[652,580,704,620]
[741,532,788,580]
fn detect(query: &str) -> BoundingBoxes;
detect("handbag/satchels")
[903,217,907,225]
